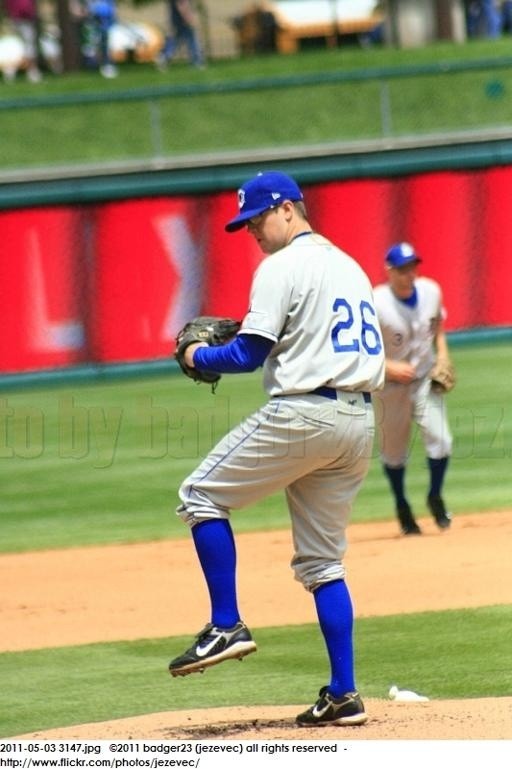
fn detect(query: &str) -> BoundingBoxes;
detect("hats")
[225,171,304,233]
[386,242,422,268]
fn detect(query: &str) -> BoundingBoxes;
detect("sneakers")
[428,495,451,527]
[169,621,257,677]
[397,508,420,535]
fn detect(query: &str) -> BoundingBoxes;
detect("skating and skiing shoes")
[296,686,368,726]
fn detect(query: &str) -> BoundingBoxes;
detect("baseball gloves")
[428,360,455,392]
[174,317,244,394]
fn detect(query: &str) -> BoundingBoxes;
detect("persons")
[169,170,386,726]
[0,0,206,83]
[373,242,455,535]
